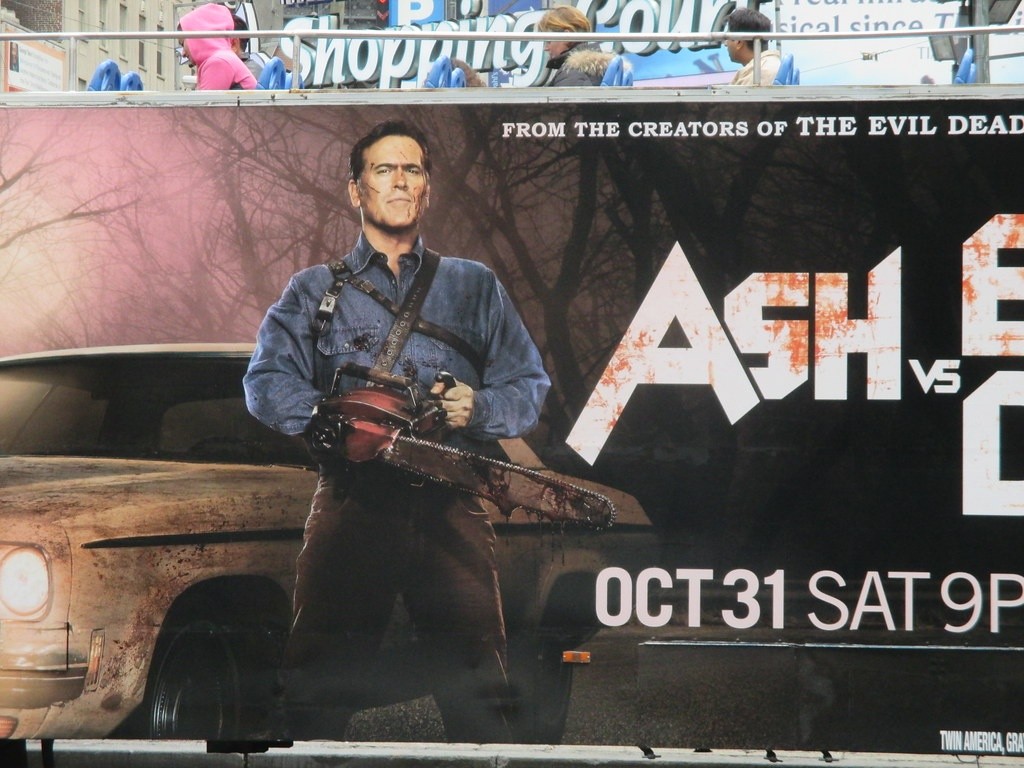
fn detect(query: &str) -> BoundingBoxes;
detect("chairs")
[82,49,978,93]
[151,393,309,469]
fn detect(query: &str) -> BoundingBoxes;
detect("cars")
[2,343,655,743]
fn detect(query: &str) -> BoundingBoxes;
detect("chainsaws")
[302,362,616,537]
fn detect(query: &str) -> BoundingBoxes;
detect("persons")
[535,6,603,86]
[177,3,257,90]
[243,121,552,744]
[230,15,264,82]
[725,8,782,85]
[451,59,486,87]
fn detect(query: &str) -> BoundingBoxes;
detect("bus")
[2,30,1023,768]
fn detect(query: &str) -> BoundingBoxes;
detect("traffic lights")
[459,0,484,18]
[376,0,390,30]
